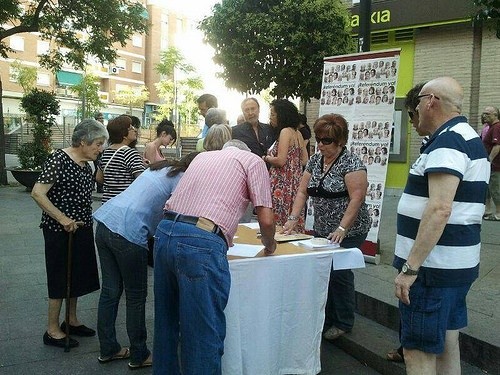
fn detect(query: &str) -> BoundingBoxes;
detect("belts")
[162,212,227,243]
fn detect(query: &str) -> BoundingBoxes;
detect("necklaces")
[322,157,335,172]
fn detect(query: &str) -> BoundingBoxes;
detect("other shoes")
[387,351,404,363]
[43,330,80,348]
[60,320,96,337]
[482,213,500,221]
[323,325,346,340]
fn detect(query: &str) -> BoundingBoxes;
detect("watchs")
[402,263,419,275]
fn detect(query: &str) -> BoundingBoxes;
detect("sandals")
[127,354,153,369]
[97,347,130,364]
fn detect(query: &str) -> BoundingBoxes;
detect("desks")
[221,222,366,375]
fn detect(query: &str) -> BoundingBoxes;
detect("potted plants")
[4,89,60,189]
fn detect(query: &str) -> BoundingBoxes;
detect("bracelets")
[338,226,348,233]
[287,216,299,221]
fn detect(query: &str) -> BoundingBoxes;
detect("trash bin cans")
[142,112,150,124]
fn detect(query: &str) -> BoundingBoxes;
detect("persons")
[393,76,491,375]
[321,61,397,107]
[365,181,382,227]
[31,119,110,348]
[92,151,200,368]
[92,113,142,207]
[279,113,372,340]
[261,99,309,234]
[480,106,500,221]
[350,121,390,166]
[142,94,311,172]
[386,80,433,362]
[152,139,276,375]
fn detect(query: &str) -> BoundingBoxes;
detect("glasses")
[127,126,135,132]
[414,93,441,103]
[258,142,267,156]
[406,110,418,120]
[315,136,335,145]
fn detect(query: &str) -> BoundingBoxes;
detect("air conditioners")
[109,67,119,74]
